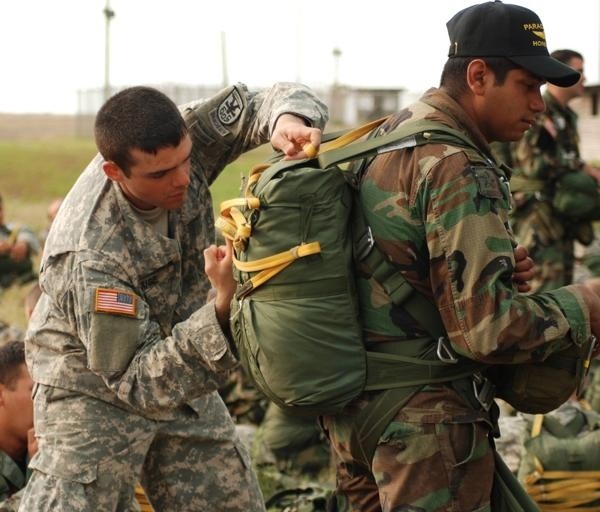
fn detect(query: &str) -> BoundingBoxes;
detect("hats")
[445,0,581,89]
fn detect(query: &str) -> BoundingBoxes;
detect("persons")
[512,49,600,293]
[18,82,332,512]
[322,0,600,512]
[1,195,600,511]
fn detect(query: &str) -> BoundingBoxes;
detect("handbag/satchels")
[551,168,600,223]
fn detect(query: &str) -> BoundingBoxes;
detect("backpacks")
[226,121,499,416]
